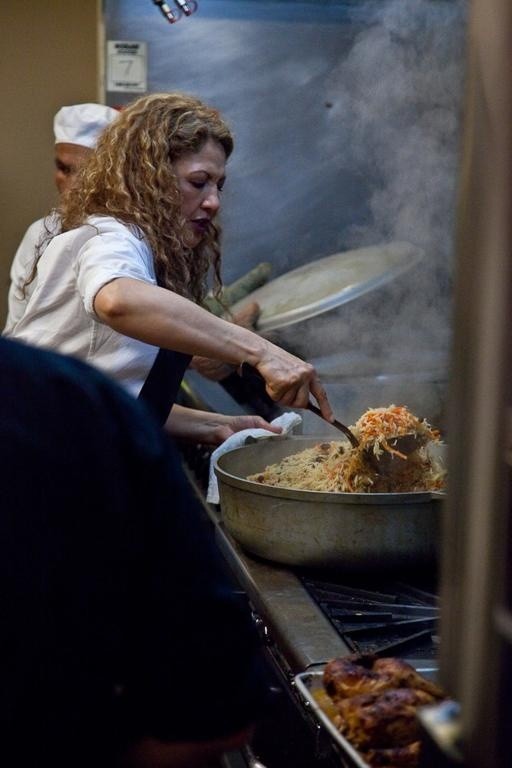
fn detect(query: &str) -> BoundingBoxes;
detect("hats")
[52,102,122,150]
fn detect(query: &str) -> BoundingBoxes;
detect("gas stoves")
[208,499,445,725]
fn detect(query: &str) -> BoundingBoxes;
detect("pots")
[215,434,446,565]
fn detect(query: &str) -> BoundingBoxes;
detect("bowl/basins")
[303,347,449,424]
[215,244,437,363]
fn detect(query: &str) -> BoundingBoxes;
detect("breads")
[323,656,448,768]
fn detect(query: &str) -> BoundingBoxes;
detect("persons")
[0,335,254,765]
[0,102,118,337]
[9,94,334,447]
